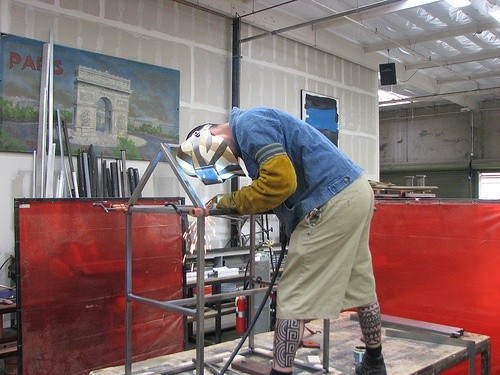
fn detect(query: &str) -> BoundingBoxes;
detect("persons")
[176,105,387,375]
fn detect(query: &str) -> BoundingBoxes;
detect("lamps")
[312,0,500,84]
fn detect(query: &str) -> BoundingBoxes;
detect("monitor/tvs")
[478,171,500,200]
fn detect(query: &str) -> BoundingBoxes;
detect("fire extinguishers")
[234,296,247,335]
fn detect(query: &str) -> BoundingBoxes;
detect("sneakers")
[355,352,387,375]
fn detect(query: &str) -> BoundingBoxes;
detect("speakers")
[379,63,396,86]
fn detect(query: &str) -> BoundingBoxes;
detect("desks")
[186,266,280,344]
[88,311,489,375]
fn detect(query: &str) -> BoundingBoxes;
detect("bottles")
[353,346,366,365]
